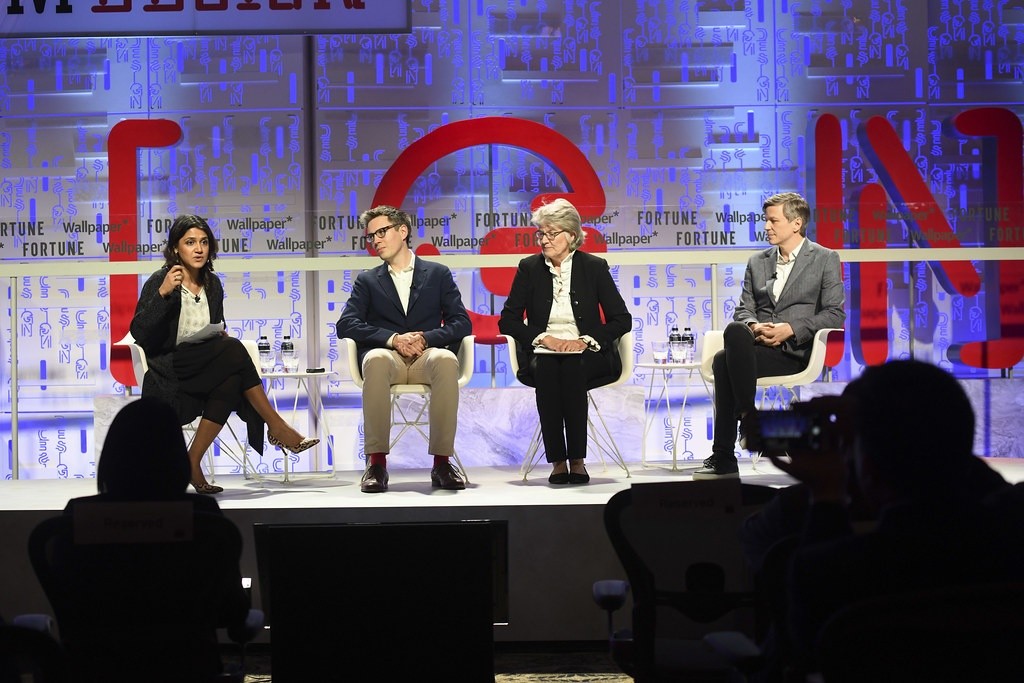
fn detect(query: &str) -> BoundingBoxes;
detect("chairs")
[25,497,252,683]
[112,330,262,484]
[497,317,633,482]
[700,328,844,470]
[593,477,782,683]
[340,334,478,486]
[820,578,1024,683]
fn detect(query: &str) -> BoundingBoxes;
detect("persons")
[692,192,847,478]
[736,357,1024,683]
[130,213,320,493]
[48,397,248,640]
[497,198,633,484]
[336,206,473,492]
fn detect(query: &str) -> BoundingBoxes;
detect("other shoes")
[548,465,569,484]
[569,466,590,485]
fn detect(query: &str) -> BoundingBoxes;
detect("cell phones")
[757,408,817,455]
[306,368,325,373]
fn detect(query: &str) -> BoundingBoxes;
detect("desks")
[242,371,337,486]
[635,362,717,473]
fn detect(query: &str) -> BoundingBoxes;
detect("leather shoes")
[361,460,389,493]
[431,460,465,490]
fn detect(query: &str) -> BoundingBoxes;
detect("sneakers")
[738,410,762,450]
[692,451,739,482]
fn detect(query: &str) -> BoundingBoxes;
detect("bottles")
[669,328,682,363]
[258,336,271,373]
[682,327,694,364]
[281,336,294,373]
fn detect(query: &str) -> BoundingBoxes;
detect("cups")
[259,350,277,374]
[671,341,688,363]
[651,341,670,364]
[281,350,301,373]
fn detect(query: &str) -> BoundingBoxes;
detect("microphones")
[775,272,777,275]
[411,282,417,288]
[195,296,200,302]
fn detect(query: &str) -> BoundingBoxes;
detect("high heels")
[189,476,223,494]
[267,430,320,456]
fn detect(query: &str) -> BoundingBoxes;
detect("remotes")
[175,259,181,290]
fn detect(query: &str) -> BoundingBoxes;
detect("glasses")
[363,224,397,243]
[535,229,565,240]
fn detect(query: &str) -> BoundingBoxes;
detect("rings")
[176,276,177,280]
